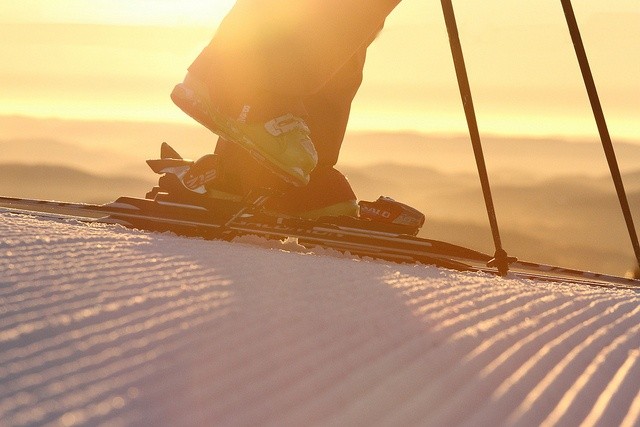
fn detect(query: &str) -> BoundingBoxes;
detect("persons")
[170,0,403,218]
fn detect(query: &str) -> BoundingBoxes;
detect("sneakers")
[159,155,359,218]
[170,47,317,184]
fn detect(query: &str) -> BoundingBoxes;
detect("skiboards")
[2,195,640,291]
